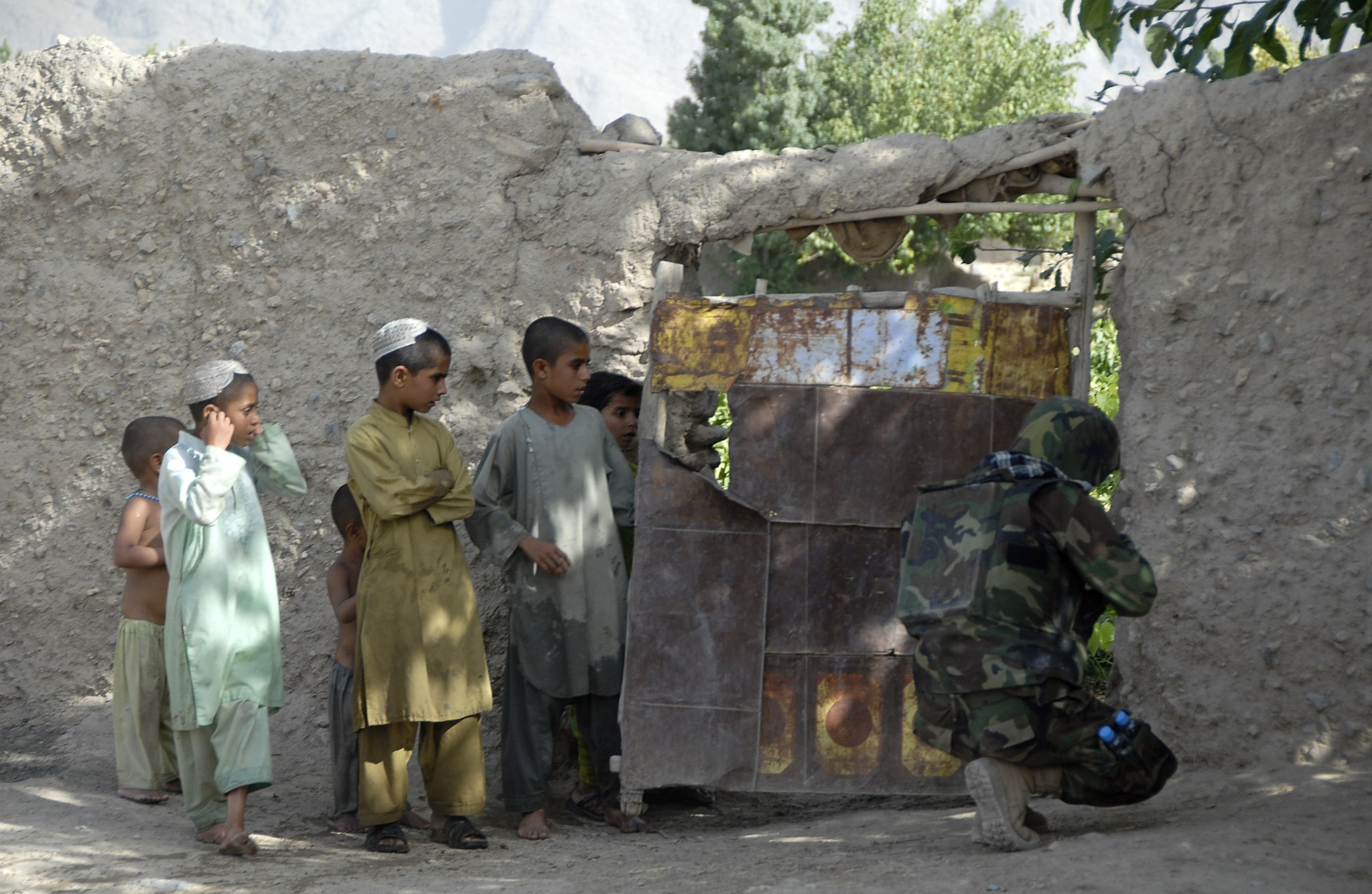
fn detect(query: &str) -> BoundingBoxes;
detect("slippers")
[431,815,489,849]
[364,822,411,853]
[566,794,606,822]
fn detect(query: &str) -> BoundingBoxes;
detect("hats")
[372,318,430,362]
[183,359,250,404]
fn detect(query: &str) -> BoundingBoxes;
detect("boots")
[969,804,1047,846]
[965,758,1061,852]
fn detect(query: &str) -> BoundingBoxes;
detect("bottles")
[1115,709,1139,737]
[1099,727,1133,760]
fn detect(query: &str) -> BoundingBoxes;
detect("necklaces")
[125,488,159,501]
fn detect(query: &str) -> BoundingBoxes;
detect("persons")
[464,316,716,839]
[112,417,188,803]
[157,358,307,853]
[909,396,1177,851]
[326,318,489,850]
